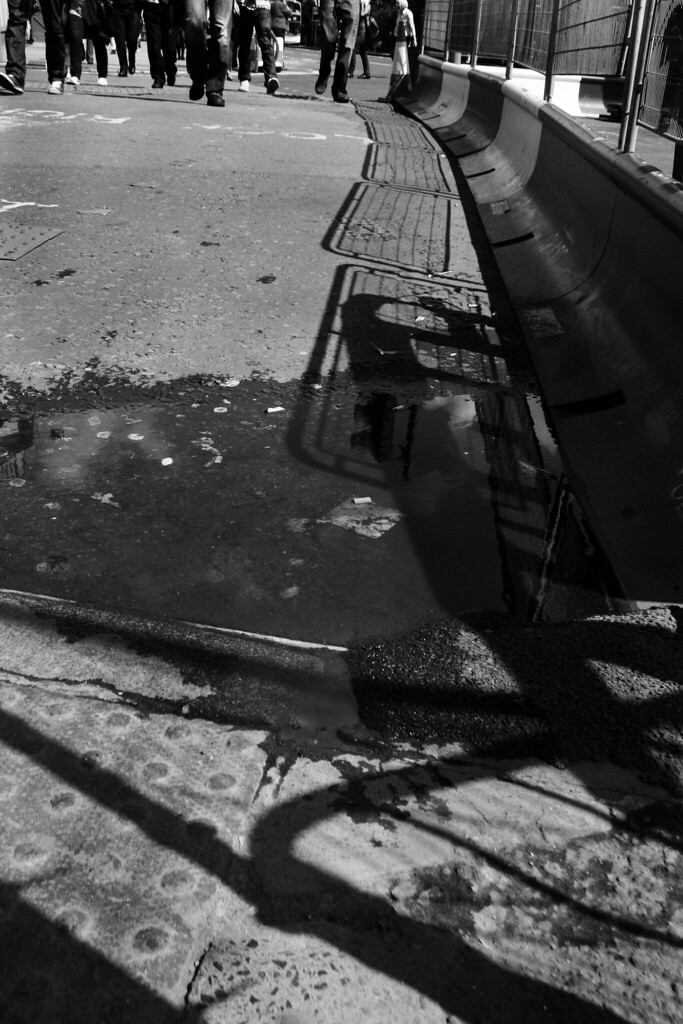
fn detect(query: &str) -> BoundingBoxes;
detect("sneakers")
[1,73,26,94]
[314,79,327,96]
[267,77,280,94]
[331,92,350,103]
[238,80,250,91]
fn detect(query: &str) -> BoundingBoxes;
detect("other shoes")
[169,68,177,85]
[66,76,79,85]
[48,81,65,94]
[151,78,166,89]
[348,73,354,78]
[188,84,203,99]
[98,77,107,86]
[117,70,128,77]
[358,75,371,79]
[208,95,226,107]
[128,66,136,74]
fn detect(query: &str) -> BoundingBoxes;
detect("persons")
[0,0,417,108]
